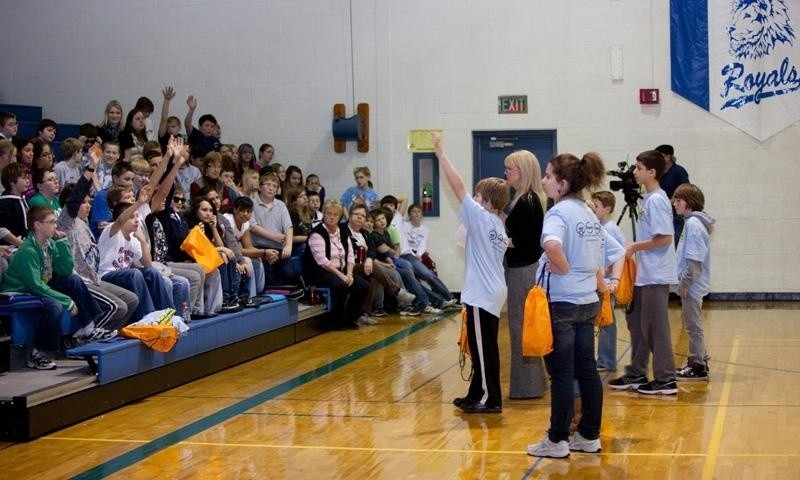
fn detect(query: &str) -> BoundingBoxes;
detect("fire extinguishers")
[423,182,433,212]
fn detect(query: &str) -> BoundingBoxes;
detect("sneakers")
[637,380,678,395]
[28,352,56,370]
[85,328,120,344]
[358,313,377,324]
[372,309,387,316]
[568,432,601,453]
[526,439,570,458]
[454,395,503,414]
[395,287,416,303]
[397,298,458,316]
[608,374,649,390]
[676,358,709,380]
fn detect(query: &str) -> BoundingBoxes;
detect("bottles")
[357,245,366,263]
[179,301,192,324]
[308,285,317,305]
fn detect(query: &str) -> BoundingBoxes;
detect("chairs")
[0,101,334,380]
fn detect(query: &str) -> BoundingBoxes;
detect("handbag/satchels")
[522,262,554,357]
[594,291,613,327]
[123,306,189,353]
[613,255,637,305]
[458,307,474,381]
[180,225,225,274]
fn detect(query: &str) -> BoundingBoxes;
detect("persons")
[0,86,457,370]
[431,130,716,460]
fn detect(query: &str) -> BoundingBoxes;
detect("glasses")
[174,197,186,204]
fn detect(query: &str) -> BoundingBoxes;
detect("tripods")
[616,202,638,243]
